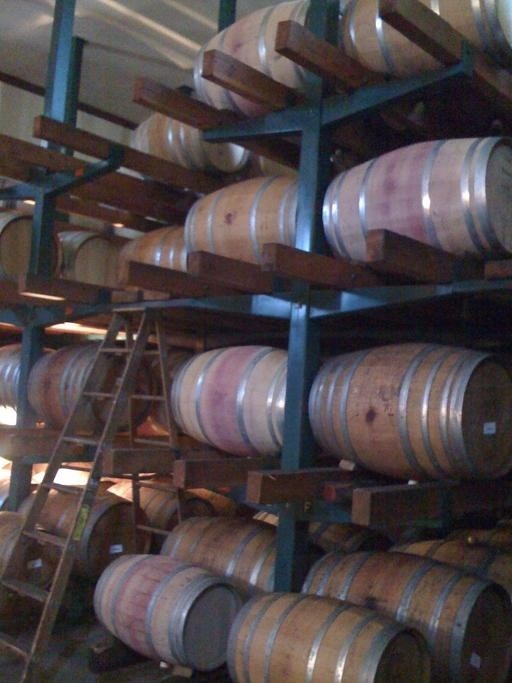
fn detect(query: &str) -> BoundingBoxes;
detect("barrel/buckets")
[1,343,57,421]
[26,340,154,433]
[170,344,288,456]
[115,225,187,301]
[138,349,197,436]
[0,525,60,616]
[17,485,154,580]
[93,553,245,673]
[185,485,262,520]
[322,136,511,263]
[106,477,216,556]
[307,341,512,483]
[130,111,251,176]
[253,506,393,556]
[159,514,326,603]
[0,208,64,287]
[193,0,344,120]
[226,592,433,683]
[56,229,121,290]
[300,548,512,682]
[446,526,511,552]
[389,537,512,599]
[183,175,300,266]
[343,0,510,80]
[0,477,63,514]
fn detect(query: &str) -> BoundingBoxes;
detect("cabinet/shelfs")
[1,2,512,682]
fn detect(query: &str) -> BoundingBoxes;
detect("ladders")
[0,307,193,683]
